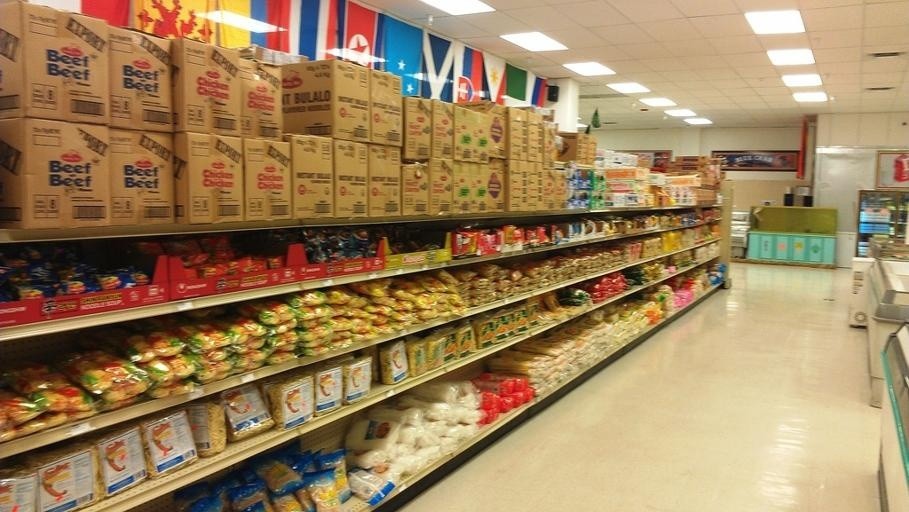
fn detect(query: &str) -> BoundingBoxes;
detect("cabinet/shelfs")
[895,190,909,260]
[1,205,724,511]
[745,231,835,266]
[854,191,897,260]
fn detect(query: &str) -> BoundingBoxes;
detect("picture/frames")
[875,149,909,192]
